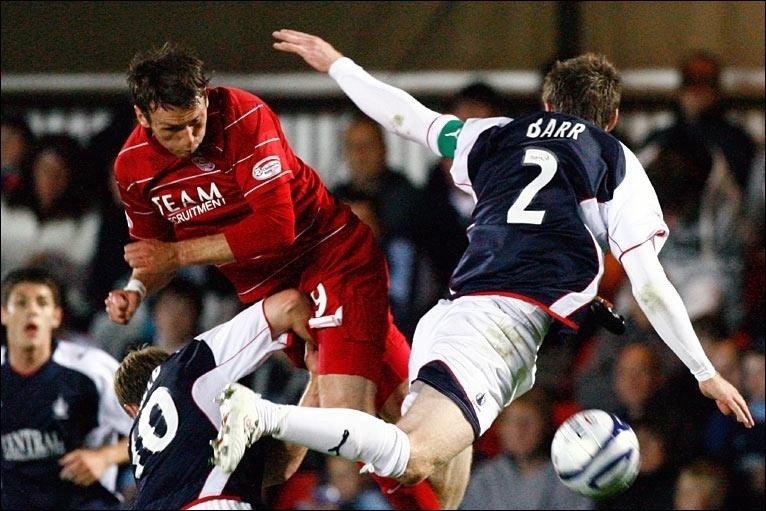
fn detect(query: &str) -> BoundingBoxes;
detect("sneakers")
[210,383,263,474]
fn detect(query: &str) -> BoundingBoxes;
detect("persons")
[273,456,393,510]
[104,44,441,510]
[461,47,766,510]
[207,27,757,511]
[330,72,517,339]
[0,103,320,509]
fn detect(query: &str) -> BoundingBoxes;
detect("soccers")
[551,409,641,498]
